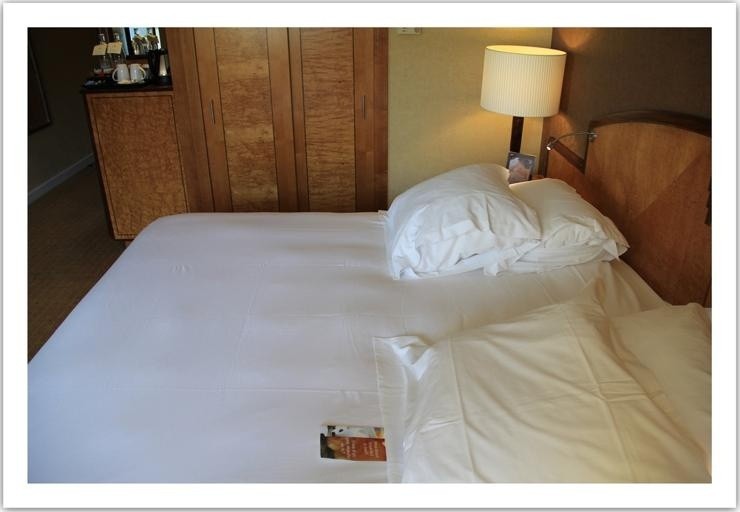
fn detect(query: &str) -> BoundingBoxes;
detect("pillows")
[377,163,542,281]
[610,303,712,476]
[509,177,631,272]
[372,278,712,484]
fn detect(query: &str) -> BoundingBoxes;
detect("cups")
[112,63,130,85]
[130,64,146,83]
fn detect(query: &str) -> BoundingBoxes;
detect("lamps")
[480,45,568,153]
[547,130,595,151]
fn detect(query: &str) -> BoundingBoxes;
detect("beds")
[28,108,712,484]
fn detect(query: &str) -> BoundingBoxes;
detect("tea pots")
[147,49,172,86]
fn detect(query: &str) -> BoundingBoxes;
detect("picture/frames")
[28,46,51,134]
[506,152,535,183]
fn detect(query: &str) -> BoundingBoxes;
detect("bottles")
[132,32,158,56]
[97,34,113,75]
[108,33,126,69]
